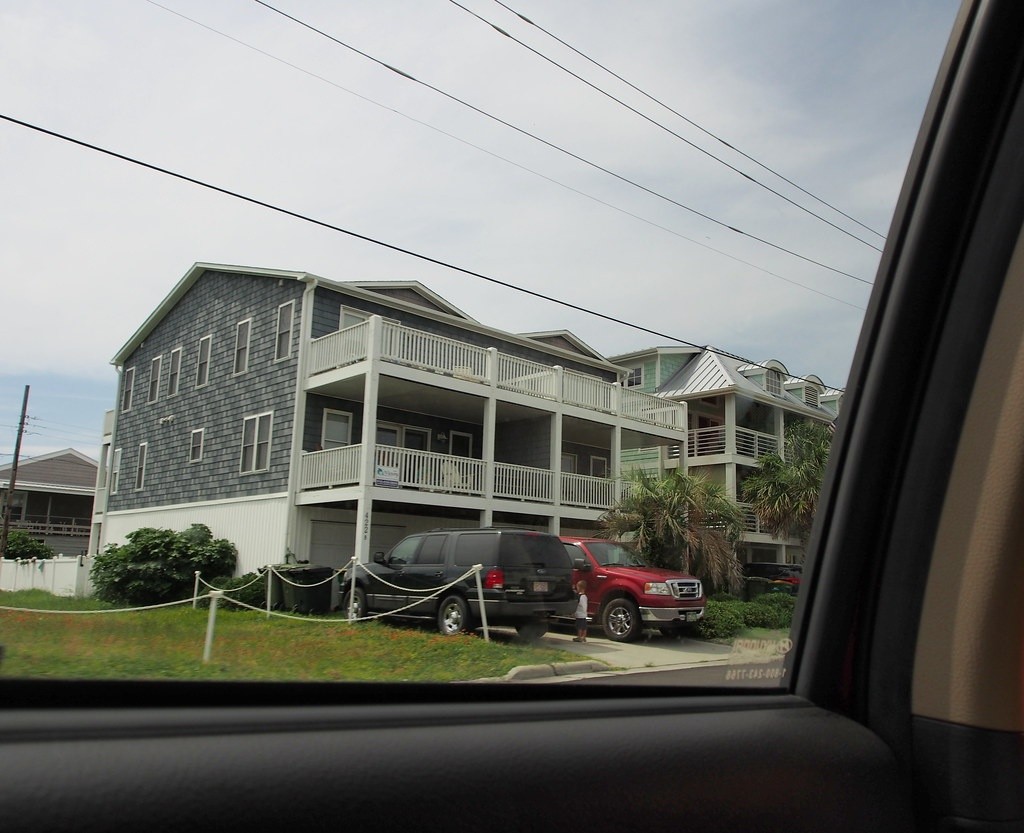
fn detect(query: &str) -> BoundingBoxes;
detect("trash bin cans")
[256,564,281,611]
[288,562,334,618]
[278,563,298,611]
[334,568,348,611]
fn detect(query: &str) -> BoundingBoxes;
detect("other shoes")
[573,637,583,642]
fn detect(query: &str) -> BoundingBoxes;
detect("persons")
[574,581,588,642]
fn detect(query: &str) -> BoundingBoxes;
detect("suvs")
[737,561,804,596]
[339,528,579,640]
[558,536,704,643]
